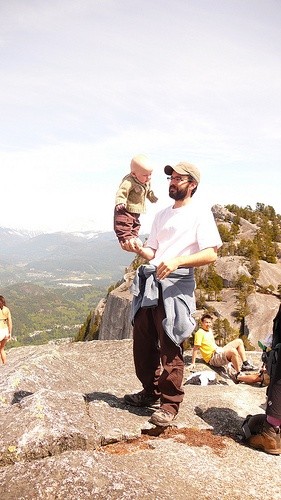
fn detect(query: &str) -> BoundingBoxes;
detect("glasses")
[167,177,191,182]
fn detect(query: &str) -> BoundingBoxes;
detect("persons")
[114,154,159,247]
[0,296,12,364]
[125,162,223,427]
[189,314,271,384]
[246,305,281,455]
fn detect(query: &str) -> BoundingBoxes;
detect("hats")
[164,163,200,185]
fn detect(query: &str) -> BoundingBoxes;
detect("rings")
[164,270,166,272]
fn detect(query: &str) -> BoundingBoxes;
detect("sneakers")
[241,363,259,371]
[246,419,281,455]
[151,408,175,426]
[124,392,161,408]
[235,372,247,383]
